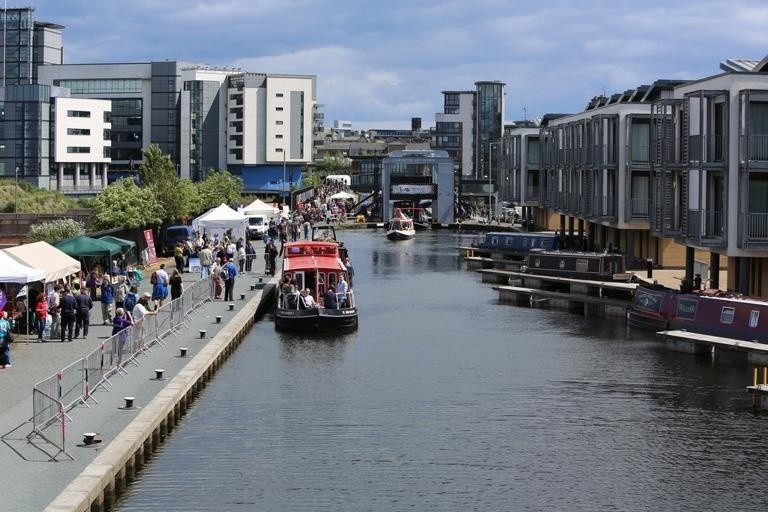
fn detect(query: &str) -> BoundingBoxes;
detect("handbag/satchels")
[47,305,60,315]
[221,269,230,281]
[6,329,14,343]
[150,272,157,285]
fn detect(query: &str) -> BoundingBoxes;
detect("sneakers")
[0,365,12,368]
[62,335,86,341]
[37,339,46,342]
[154,304,157,310]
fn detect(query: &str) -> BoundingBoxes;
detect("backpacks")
[116,285,124,302]
[125,293,136,311]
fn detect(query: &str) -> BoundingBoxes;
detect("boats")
[274,226,358,331]
[456,229,627,283]
[625,284,768,342]
[386,217,415,240]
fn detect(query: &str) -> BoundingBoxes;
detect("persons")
[1,170,348,366]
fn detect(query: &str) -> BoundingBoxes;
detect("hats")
[143,293,152,298]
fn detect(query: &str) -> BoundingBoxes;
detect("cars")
[165,226,196,256]
